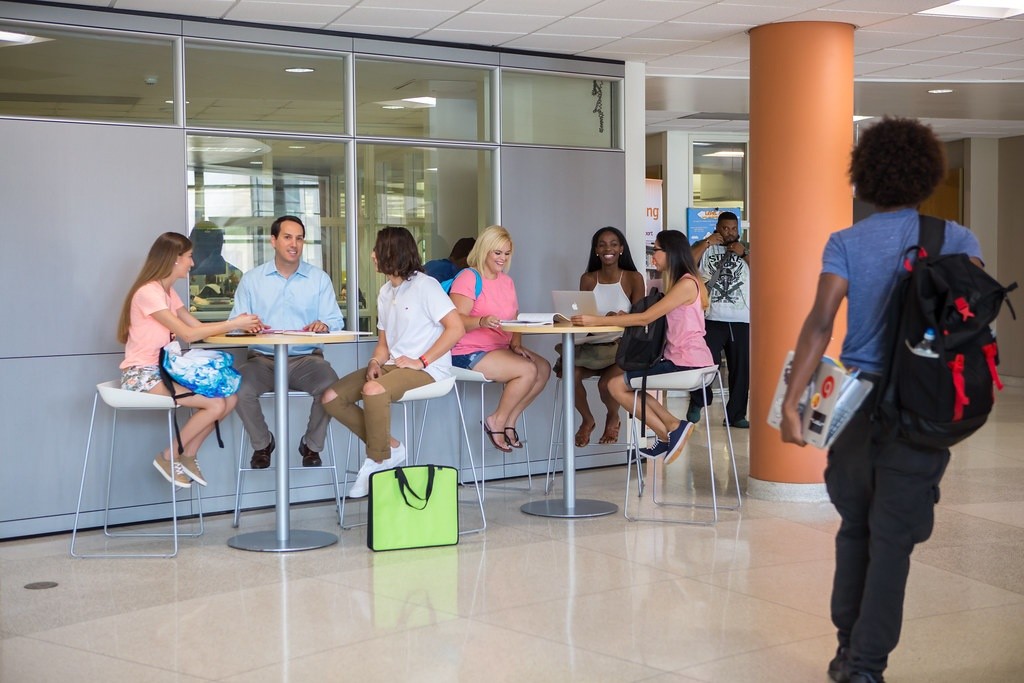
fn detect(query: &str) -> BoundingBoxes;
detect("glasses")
[652,248,664,253]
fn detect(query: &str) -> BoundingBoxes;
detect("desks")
[203,331,357,552]
[500,325,626,519]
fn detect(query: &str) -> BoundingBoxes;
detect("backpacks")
[440,268,482,299]
[615,287,666,370]
[874,215,1018,448]
[159,340,243,398]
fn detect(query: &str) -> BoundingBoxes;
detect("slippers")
[575,422,595,448]
[600,421,621,444]
[505,427,523,448]
[480,420,512,452]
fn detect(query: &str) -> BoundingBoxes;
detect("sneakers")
[664,420,694,464]
[349,456,398,499]
[153,456,191,489]
[639,439,671,459]
[391,441,406,464]
[179,452,207,487]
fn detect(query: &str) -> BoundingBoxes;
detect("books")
[499,313,571,326]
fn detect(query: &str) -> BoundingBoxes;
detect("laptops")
[765,351,874,451]
[552,290,598,320]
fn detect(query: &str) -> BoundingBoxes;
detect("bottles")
[914,329,935,354]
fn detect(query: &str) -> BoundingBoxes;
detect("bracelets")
[740,248,749,258]
[419,355,428,369]
[479,316,483,328]
[704,239,710,248]
[368,358,380,366]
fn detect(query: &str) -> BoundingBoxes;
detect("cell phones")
[226,333,256,336]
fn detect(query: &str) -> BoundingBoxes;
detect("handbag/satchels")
[368,464,460,552]
[576,342,619,369]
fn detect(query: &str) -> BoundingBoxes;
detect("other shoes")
[830,646,852,683]
[724,417,749,428]
[687,399,701,423]
[851,672,885,683]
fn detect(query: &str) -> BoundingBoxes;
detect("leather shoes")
[251,431,276,469]
[299,436,322,467]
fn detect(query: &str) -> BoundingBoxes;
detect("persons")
[686,211,750,429]
[228,215,345,470]
[779,112,985,683]
[553,226,645,447]
[607,230,715,465]
[421,237,476,283]
[341,286,379,336]
[319,226,467,498]
[116,232,259,489]
[449,224,551,453]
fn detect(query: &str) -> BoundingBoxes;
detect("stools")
[413,366,532,505]
[340,373,486,534]
[543,376,645,498]
[71,378,203,558]
[233,389,343,528]
[623,365,741,524]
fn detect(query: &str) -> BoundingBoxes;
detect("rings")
[731,243,733,248]
